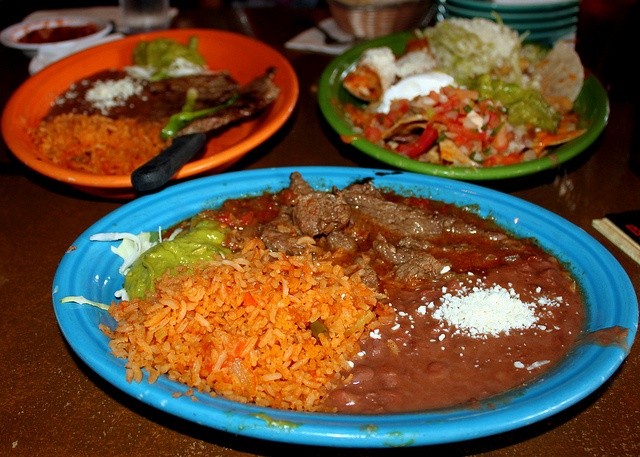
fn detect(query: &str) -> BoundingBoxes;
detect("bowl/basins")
[328,1,434,40]
[0,13,114,57]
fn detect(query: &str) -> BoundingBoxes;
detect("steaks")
[43,64,277,137]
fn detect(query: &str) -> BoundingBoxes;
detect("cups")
[117,0,170,35]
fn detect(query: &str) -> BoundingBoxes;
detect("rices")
[97,247,392,415]
[26,113,170,175]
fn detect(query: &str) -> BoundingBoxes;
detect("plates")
[438,1,578,54]
[0,28,301,203]
[22,6,179,34]
[317,27,612,184]
[51,165,638,449]
[284,15,352,57]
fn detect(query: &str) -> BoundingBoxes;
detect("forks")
[314,24,339,44]
